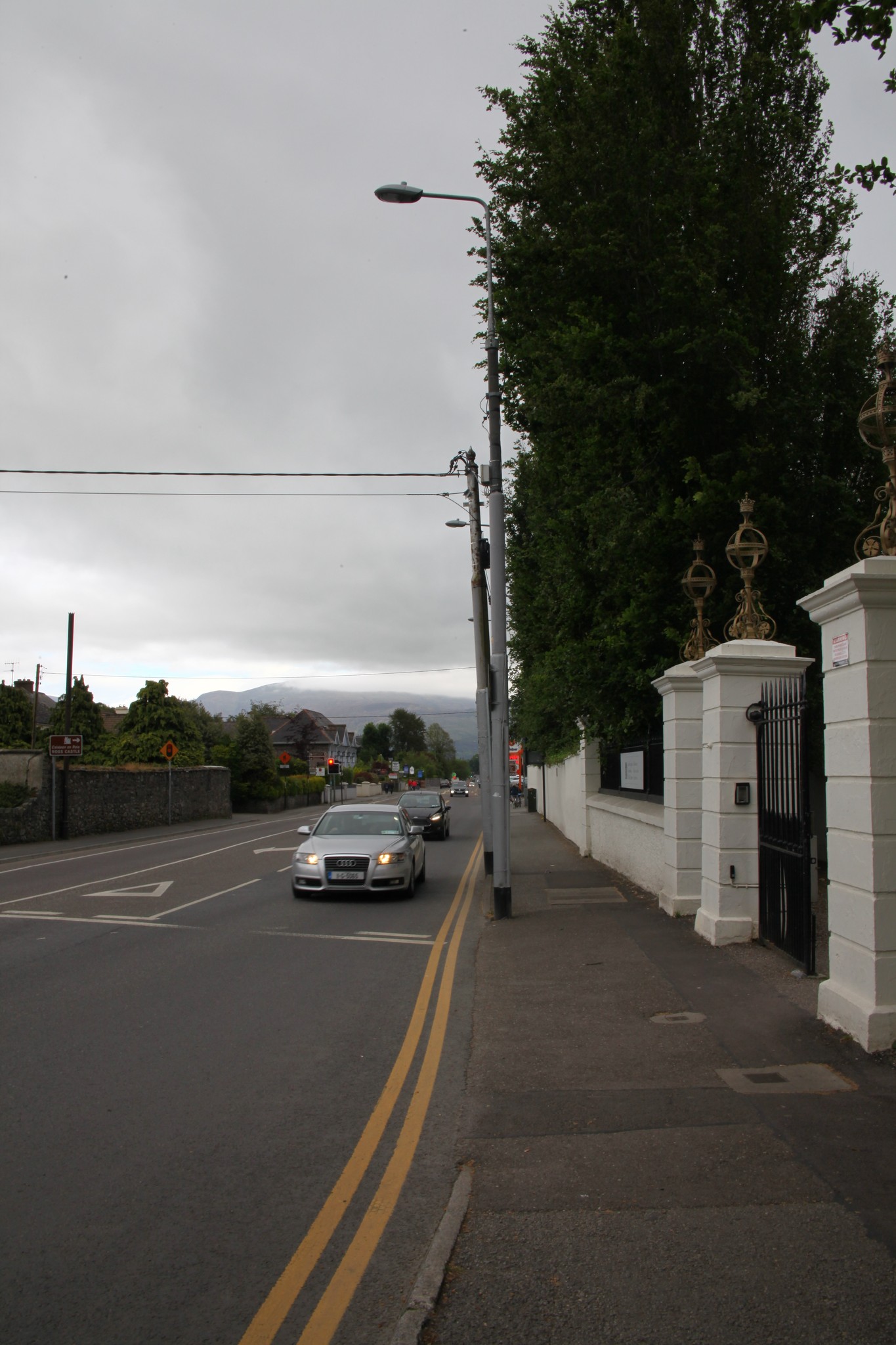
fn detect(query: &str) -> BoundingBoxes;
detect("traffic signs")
[49,735,83,757]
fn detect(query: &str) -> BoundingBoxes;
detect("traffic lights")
[327,758,340,775]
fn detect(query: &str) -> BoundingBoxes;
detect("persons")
[389,780,394,794]
[510,783,519,803]
[384,781,389,794]
[408,779,420,791]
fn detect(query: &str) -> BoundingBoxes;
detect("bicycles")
[511,793,522,809]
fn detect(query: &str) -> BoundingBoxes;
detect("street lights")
[373,179,516,927]
[443,517,515,922]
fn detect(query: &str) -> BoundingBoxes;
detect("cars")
[509,777,523,785]
[469,782,475,787]
[473,776,476,778]
[396,791,452,841]
[290,804,427,901]
[439,779,470,789]
[472,779,475,782]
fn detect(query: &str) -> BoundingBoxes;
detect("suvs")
[449,781,469,797]
